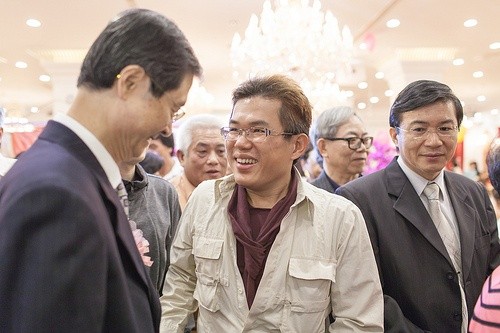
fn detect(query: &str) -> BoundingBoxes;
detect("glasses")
[395,125,460,138]
[117,71,185,123]
[324,137,374,151]
[220,126,293,143]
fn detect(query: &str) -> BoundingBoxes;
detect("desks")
[2,121,44,160]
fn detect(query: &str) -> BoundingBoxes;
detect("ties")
[116,179,130,222]
[424,184,469,333]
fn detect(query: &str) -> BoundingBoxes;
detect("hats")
[305,142,314,154]
[157,131,175,147]
[139,149,165,175]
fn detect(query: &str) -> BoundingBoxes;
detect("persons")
[469,136,500,333]
[172,114,233,214]
[309,106,369,193]
[139,130,322,185]
[159,74,384,333]
[335,80,500,333]
[117,148,182,298]
[0,10,203,333]
